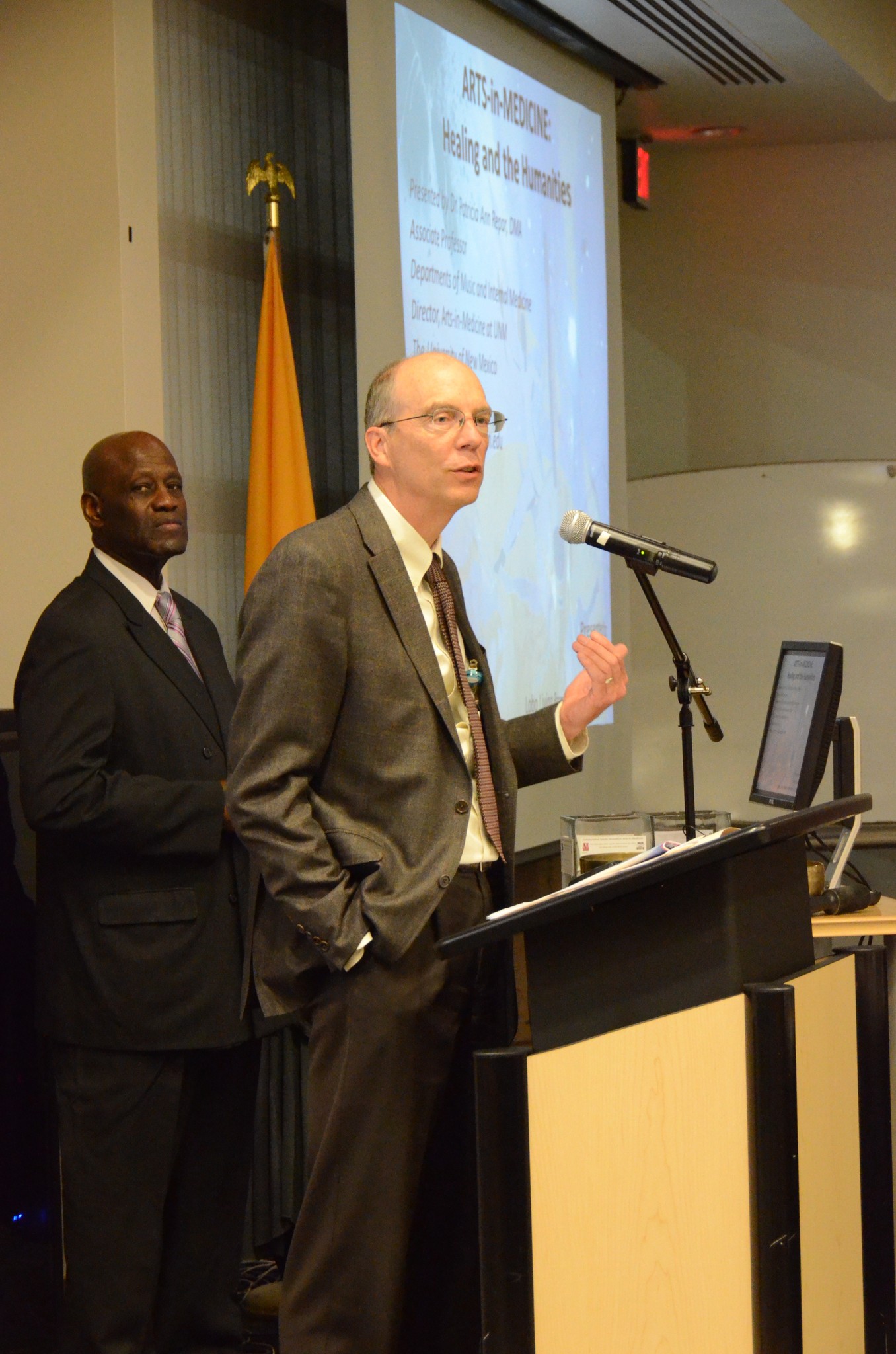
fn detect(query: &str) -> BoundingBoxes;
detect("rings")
[605,676,613,684]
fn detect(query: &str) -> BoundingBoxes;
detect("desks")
[806,882,893,1190]
[433,794,895,1354]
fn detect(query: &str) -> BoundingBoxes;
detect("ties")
[424,553,508,865]
[154,592,205,685]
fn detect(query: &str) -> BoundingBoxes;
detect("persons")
[225,346,629,1354]
[12,431,260,1354]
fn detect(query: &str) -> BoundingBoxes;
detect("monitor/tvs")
[748,640,862,890]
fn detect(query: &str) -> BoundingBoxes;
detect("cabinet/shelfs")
[559,808,731,892]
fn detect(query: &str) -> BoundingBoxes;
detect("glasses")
[379,407,508,433]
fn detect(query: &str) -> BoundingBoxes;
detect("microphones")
[558,509,718,584]
